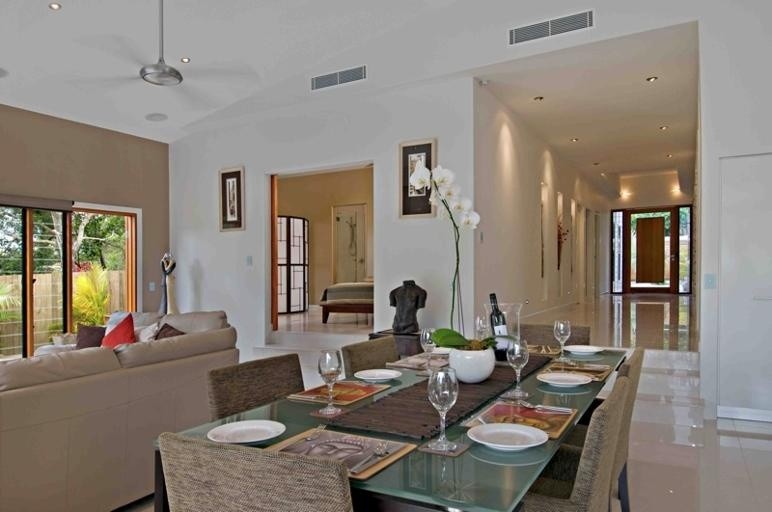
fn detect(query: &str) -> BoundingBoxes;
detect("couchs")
[0,311,239,512]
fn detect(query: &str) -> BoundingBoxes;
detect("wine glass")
[318,350,342,414]
[476,314,490,339]
[427,367,459,451]
[420,328,436,353]
[553,319,571,361]
[506,339,529,397]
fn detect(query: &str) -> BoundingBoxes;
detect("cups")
[483,304,523,367]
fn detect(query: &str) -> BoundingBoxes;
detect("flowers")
[409,159,481,337]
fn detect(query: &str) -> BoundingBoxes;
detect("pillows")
[76,313,186,351]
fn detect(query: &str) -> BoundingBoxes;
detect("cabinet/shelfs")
[344,210,357,281]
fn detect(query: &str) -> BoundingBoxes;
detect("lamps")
[139,0,183,85]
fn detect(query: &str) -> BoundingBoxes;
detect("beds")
[319,274,373,324]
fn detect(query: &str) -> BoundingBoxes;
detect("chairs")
[206,353,305,423]
[562,346,645,512]
[513,376,633,512]
[514,324,590,346]
[157,432,354,512]
[341,335,401,378]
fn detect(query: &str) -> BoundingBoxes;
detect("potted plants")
[47,322,63,345]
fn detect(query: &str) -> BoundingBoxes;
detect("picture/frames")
[218,165,245,231]
[398,137,437,219]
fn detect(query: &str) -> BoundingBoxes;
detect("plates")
[467,422,549,450]
[567,354,604,361]
[434,346,451,355]
[206,420,286,443]
[354,369,401,382]
[536,382,592,395]
[536,373,592,387]
[467,442,551,467]
[565,343,604,356]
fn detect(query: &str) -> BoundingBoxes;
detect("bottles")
[489,293,508,361]
